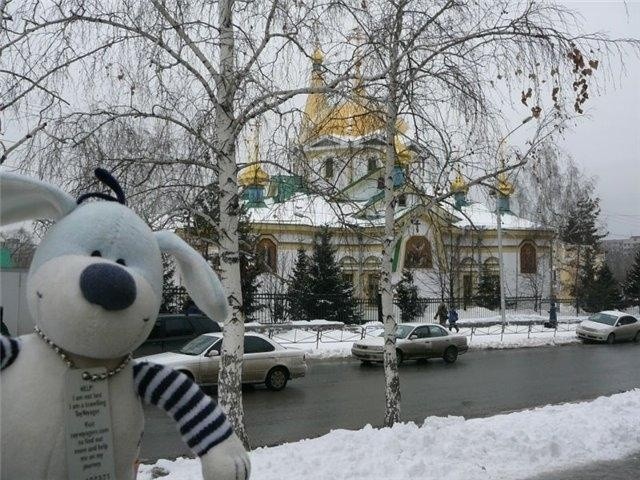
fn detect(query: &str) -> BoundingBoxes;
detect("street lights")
[495,117,534,323]
[292,211,316,253]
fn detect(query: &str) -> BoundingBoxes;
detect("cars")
[575,310,640,344]
[351,323,469,366]
[132,313,221,357]
[138,332,307,392]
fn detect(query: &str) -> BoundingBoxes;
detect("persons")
[446,306,460,334]
[433,301,448,329]
[547,303,558,327]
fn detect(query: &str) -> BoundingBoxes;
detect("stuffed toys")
[0,164,253,480]
